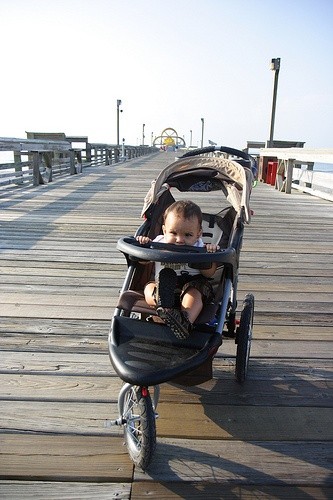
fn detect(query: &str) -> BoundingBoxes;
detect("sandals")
[156,268,177,309]
[158,306,195,341]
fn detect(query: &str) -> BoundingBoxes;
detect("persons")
[175,144,179,153]
[165,144,168,152]
[136,201,220,340]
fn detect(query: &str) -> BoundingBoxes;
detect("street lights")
[143,124,145,145]
[269,57,281,148]
[116,99,121,145]
[190,130,192,147]
[201,118,204,148]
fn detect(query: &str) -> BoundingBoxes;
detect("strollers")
[108,145,255,469]
